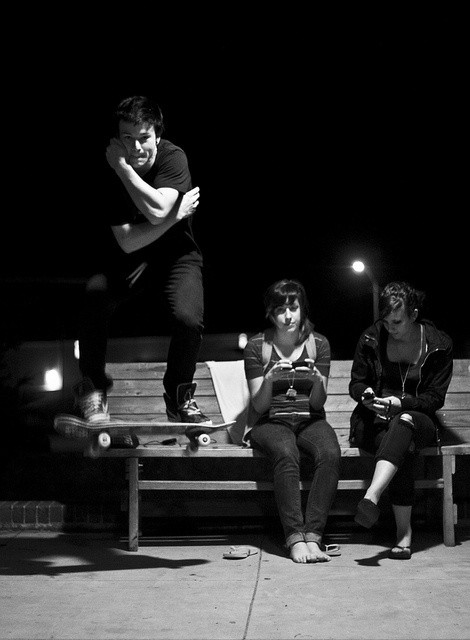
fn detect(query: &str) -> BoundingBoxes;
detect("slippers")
[354,499,379,529]
[321,543,343,556]
[389,545,414,560]
[223,546,260,558]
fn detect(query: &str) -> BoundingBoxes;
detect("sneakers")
[162,383,213,425]
[82,391,110,424]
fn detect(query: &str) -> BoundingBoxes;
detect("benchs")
[85,358,470,552]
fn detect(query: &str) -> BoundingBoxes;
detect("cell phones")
[361,392,374,399]
[283,361,308,372]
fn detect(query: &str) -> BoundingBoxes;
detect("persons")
[64,96,208,424]
[227,279,341,564]
[349,282,453,558]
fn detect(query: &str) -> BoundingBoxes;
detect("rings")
[385,406,390,413]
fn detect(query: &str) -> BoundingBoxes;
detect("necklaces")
[399,324,420,400]
[286,374,297,397]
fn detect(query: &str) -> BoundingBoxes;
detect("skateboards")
[53,414,237,450]
